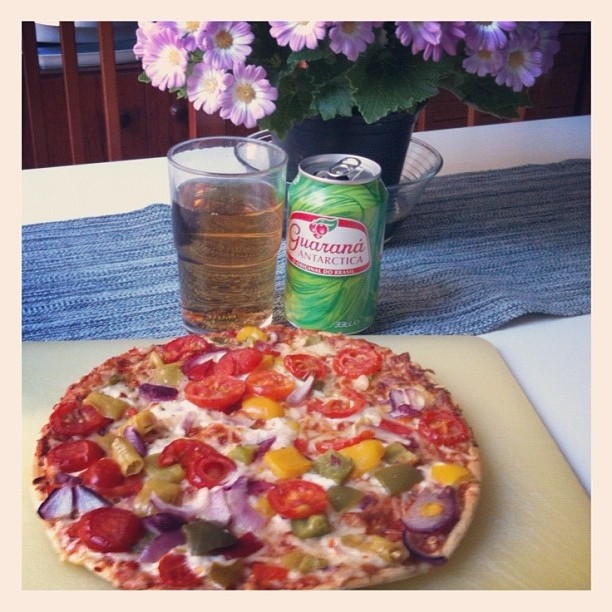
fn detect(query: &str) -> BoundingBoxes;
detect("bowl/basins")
[235,126,442,245]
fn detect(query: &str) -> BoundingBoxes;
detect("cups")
[167,135,288,335]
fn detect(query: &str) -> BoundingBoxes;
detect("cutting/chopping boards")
[21,336,591,590]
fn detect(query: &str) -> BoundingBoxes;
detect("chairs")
[21,21,227,169]
[417,93,530,132]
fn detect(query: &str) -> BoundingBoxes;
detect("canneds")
[285,153,387,335]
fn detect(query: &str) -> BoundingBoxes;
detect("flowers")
[135,20,564,141]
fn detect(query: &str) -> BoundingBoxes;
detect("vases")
[272,95,415,211]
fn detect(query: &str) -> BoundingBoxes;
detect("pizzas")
[32,326,480,591]
